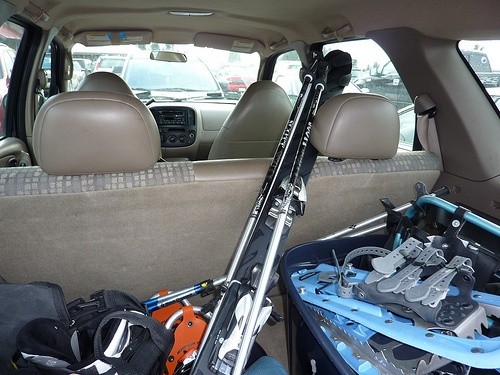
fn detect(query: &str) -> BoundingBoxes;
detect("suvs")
[355,49,494,112]
[0,43,24,136]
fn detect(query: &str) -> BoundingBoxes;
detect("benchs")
[0,71,444,307]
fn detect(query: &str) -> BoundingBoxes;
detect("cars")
[211,63,259,100]
[120,52,224,99]
[94,54,125,76]
[397,85,500,154]
[273,60,362,97]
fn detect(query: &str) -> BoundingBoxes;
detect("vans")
[72,59,92,74]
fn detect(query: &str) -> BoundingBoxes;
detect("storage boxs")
[279,229,391,375]
